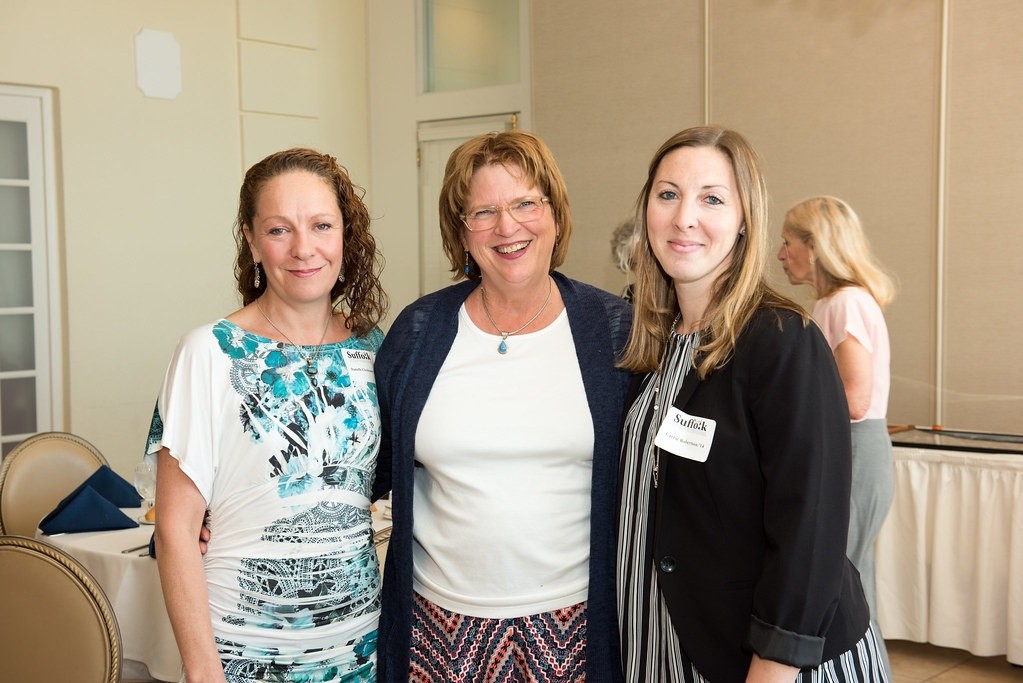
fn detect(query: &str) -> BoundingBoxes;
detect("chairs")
[0,431,111,538]
[0,534,124,683]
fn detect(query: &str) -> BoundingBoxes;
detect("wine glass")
[134,459,157,511]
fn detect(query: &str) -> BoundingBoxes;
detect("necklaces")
[254,299,333,386]
[480,277,552,354]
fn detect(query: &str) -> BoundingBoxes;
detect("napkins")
[38,485,140,538]
[59,464,144,509]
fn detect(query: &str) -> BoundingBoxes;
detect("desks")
[874,429,1023,667]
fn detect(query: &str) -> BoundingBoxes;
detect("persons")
[148,132,651,683]
[777,196,894,683]
[612,126,888,683]
[610,216,643,302]
[143,149,388,683]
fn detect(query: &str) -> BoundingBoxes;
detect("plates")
[138,516,155,524]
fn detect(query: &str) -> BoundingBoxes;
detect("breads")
[145,505,155,522]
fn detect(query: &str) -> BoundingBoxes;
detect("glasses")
[459,195,552,232]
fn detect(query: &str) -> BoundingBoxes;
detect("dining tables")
[37,491,392,683]
